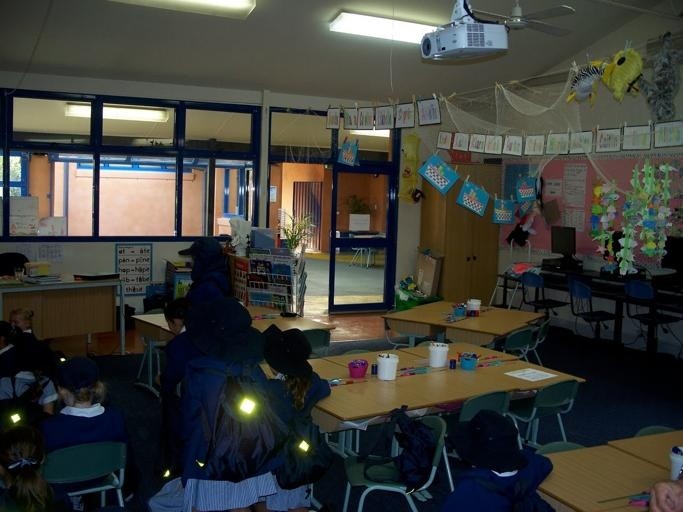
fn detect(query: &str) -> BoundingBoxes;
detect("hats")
[55,355,101,390]
[262,328,313,379]
[184,286,252,337]
[178,237,223,258]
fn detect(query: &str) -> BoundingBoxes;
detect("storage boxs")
[36,215,69,241]
[9,215,38,237]
[9,193,40,217]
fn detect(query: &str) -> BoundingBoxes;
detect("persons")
[44,355,128,512]
[160,296,193,400]
[643,477,682,511]
[147,296,278,512]
[0,426,61,511]
[0,349,58,415]
[255,327,333,511]
[155,299,191,390]
[436,408,558,511]
[0,319,48,374]
[7,307,35,334]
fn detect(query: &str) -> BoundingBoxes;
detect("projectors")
[421,24,508,62]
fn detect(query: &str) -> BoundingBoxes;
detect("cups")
[454,306,465,317]
[14,268,23,279]
[348,358,367,378]
[460,355,477,370]
[670,453,682,481]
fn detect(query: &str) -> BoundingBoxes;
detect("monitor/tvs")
[551,225,577,259]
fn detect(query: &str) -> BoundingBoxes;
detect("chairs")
[523,318,552,367]
[566,276,617,345]
[502,327,533,362]
[498,267,682,313]
[633,424,677,438]
[0,252,31,276]
[341,415,448,512]
[42,440,129,509]
[520,271,573,321]
[348,234,375,268]
[623,278,683,359]
[448,389,523,451]
[514,379,579,450]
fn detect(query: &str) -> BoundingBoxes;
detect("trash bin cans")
[115,304,136,331]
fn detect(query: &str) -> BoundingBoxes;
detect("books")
[218,227,305,312]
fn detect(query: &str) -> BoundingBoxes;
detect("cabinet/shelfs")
[415,159,505,308]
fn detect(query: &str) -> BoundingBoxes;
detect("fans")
[469,0,578,37]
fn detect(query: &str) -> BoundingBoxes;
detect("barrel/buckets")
[669,447,683,482]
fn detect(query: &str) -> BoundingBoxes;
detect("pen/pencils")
[250,313,277,320]
[477,355,504,367]
[379,352,390,359]
[672,446,683,456]
[395,365,433,377]
[445,315,467,323]
[432,341,447,347]
[328,377,368,387]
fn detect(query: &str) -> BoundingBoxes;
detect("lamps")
[349,125,390,139]
[323,8,450,47]
[109,0,256,26]
[61,100,168,125]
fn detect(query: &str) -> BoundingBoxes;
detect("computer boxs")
[542,259,584,273]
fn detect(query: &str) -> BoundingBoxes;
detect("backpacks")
[203,366,288,484]
[275,377,334,489]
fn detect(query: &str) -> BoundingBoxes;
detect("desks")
[605,430,682,470]
[333,236,386,269]
[380,300,495,353]
[336,231,380,237]
[130,302,337,398]
[440,308,548,348]
[305,343,587,460]
[535,444,679,512]
[1,275,121,360]
